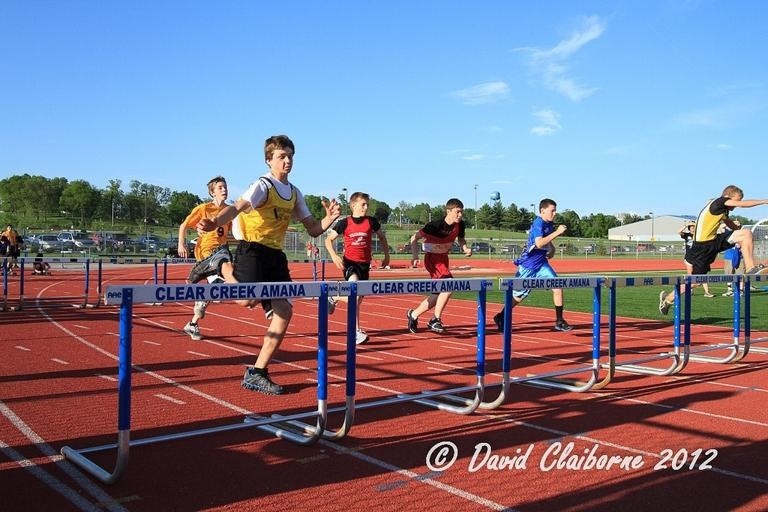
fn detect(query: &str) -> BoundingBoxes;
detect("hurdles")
[243,276,497,441]
[61,281,339,485]
[526,273,685,388]
[620,265,741,374]
[422,267,606,408]
[670,269,768,363]
[0,245,351,311]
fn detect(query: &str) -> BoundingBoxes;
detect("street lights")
[142,189,147,236]
[473,186,478,230]
[530,204,536,222]
[649,212,654,245]
[342,188,347,218]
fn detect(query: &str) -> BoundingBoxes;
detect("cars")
[60,209,71,216]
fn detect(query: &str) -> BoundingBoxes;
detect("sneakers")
[183,321,202,341]
[189,245,233,284]
[356,330,369,345]
[427,318,446,333]
[747,265,768,275]
[329,297,335,315]
[659,291,671,315]
[407,309,418,333]
[555,320,575,332]
[244,367,285,395]
[494,313,504,331]
[704,292,716,298]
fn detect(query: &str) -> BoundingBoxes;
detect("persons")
[678,221,716,297]
[0,223,52,277]
[305,239,312,257]
[324,193,390,345]
[177,176,235,340]
[405,198,472,333]
[186,135,341,396]
[311,243,319,258]
[492,199,574,332]
[718,219,747,298]
[658,184,767,316]
